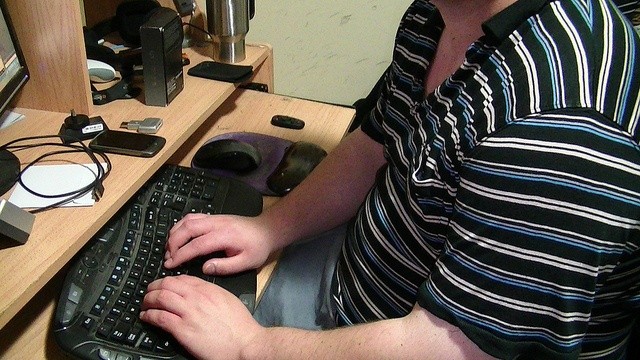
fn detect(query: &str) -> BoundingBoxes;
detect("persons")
[140,0,639,360]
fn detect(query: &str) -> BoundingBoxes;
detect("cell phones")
[86,129,168,159]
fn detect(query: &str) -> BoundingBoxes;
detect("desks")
[0,42,358,358]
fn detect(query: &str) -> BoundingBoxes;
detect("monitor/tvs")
[0,1,31,198]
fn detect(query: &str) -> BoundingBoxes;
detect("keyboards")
[45,163,265,360]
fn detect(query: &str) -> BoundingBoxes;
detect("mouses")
[189,139,263,174]
